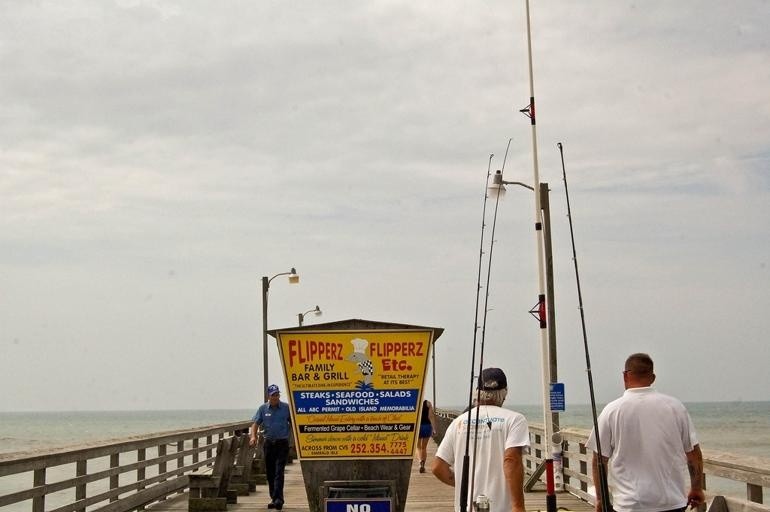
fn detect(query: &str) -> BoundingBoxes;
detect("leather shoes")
[267,499,275,509]
[274,498,283,510]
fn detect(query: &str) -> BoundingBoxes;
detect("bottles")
[472,494,491,511]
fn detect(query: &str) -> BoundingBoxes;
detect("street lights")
[486,166,564,432]
[298,304,328,326]
[262,264,301,407]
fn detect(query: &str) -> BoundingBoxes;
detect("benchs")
[187,428,269,512]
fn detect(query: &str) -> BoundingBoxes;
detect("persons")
[462,399,477,414]
[431,368,531,512]
[416,399,437,473]
[584,353,705,512]
[249,385,296,510]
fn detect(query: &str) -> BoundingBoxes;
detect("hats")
[476,367,508,392]
[266,384,281,396]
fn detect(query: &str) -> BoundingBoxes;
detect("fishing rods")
[557,142,613,512]
[461,139,513,512]
